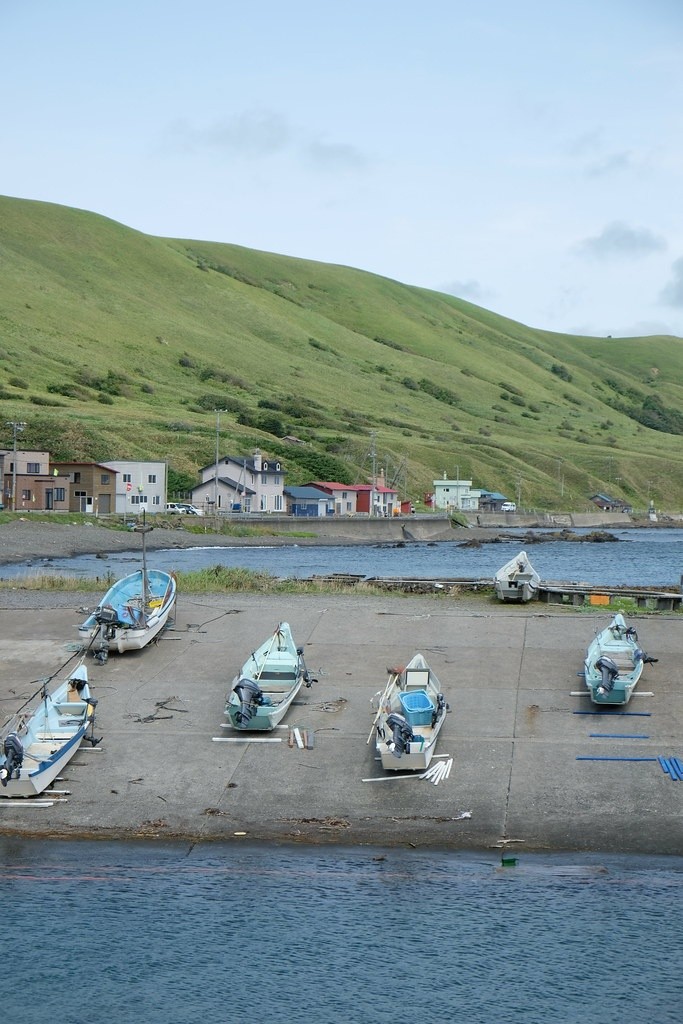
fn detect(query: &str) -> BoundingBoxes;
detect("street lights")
[214,408,228,515]
[5,422,27,512]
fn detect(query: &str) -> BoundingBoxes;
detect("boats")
[487,550,540,603]
[0,666,94,797]
[584,611,646,704]
[224,619,306,730]
[372,651,448,772]
[79,568,175,652]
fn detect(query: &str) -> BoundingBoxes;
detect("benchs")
[537,586,683,611]
[259,686,289,692]
[36,733,74,739]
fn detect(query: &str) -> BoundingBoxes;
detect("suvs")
[166,502,204,517]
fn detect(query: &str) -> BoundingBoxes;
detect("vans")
[500,501,516,514]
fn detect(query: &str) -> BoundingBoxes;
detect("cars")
[410,504,415,514]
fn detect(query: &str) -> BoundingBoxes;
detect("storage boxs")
[398,689,435,727]
[590,595,610,605]
